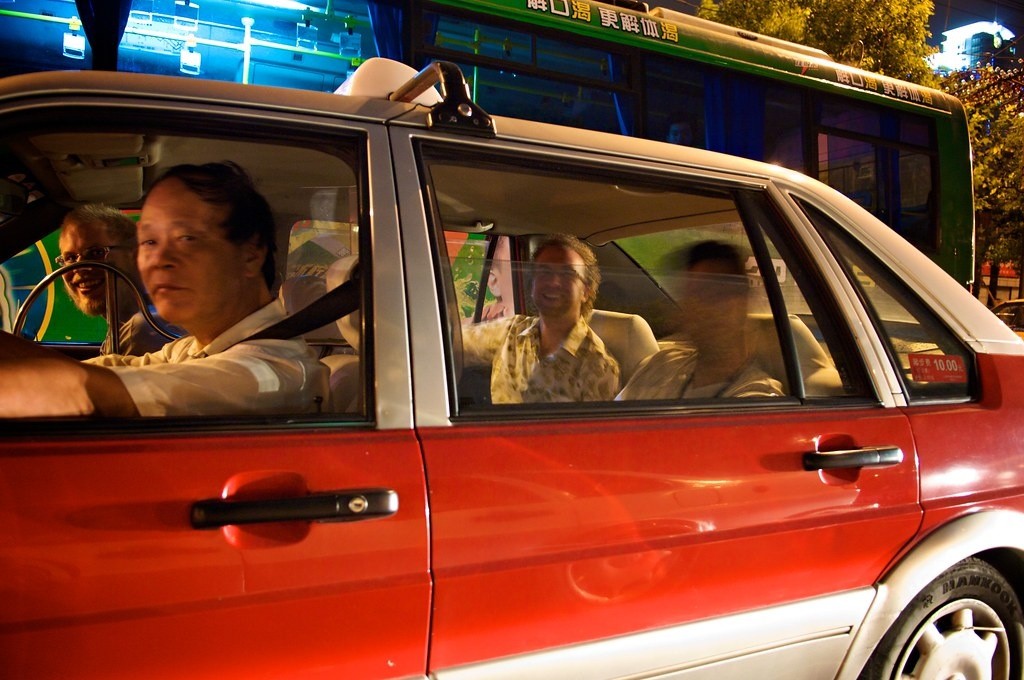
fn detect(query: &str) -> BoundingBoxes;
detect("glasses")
[54,245,120,267]
[529,264,588,289]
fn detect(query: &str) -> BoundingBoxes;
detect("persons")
[55,202,189,356]
[0,158,333,418]
[461,233,621,404]
[461,236,515,325]
[613,241,785,401]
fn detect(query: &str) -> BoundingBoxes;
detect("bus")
[1,0,976,333]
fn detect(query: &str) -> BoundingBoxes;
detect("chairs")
[276,253,367,421]
[584,310,660,386]
[744,312,845,397]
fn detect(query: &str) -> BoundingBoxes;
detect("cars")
[0,52,1024,680]
[991,298,1024,340]
